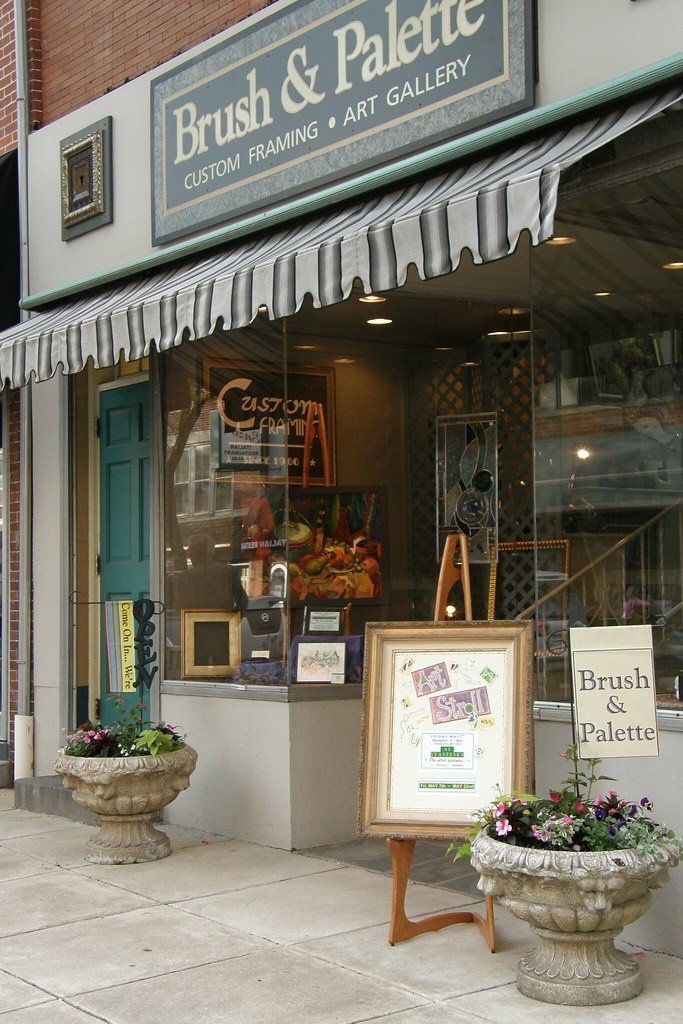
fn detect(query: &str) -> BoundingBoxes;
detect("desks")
[286,635,364,683]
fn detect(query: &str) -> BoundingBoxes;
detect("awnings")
[0,84,683,397]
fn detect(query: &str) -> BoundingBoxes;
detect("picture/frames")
[180,608,242,678]
[56,130,104,228]
[230,482,393,608]
[202,358,338,488]
[356,620,535,843]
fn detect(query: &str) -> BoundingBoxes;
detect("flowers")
[55,690,187,757]
[443,743,676,863]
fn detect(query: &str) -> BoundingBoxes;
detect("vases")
[470,826,683,1007]
[54,744,199,865]
